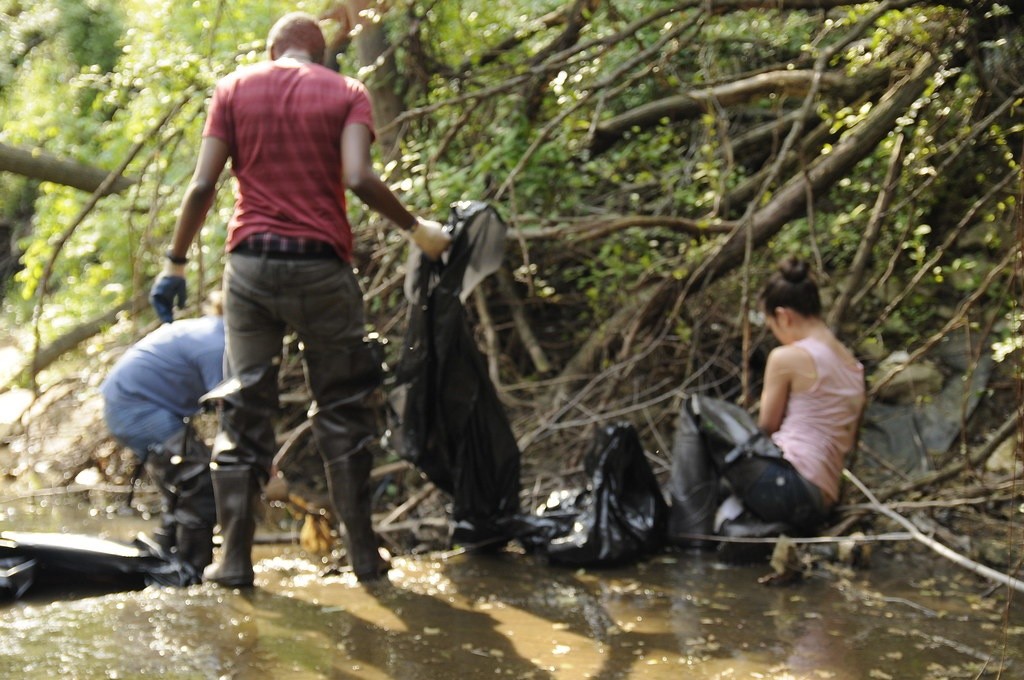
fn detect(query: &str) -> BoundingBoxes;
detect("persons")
[662,256,865,556]
[149,13,452,588]
[99,317,224,578]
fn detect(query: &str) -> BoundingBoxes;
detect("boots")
[325,442,391,580]
[202,461,258,586]
[142,434,219,583]
[649,386,777,546]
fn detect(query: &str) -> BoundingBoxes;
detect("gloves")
[410,216,451,260]
[148,249,190,324]
